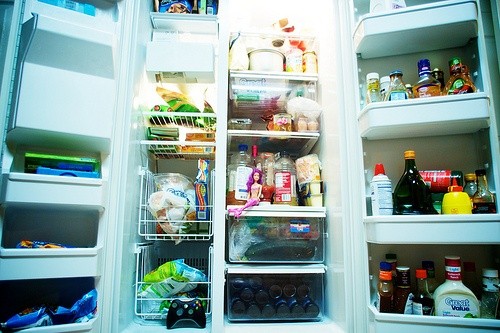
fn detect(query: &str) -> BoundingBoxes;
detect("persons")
[228,168,263,218]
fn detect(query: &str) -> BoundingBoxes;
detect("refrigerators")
[1,0,500,333]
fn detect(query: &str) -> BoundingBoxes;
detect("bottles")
[394,150,432,215]
[256,149,275,206]
[274,150,299,206]
[227,144,254,206]
[226,274,320,319]
[441,168,496,214]
[365,56,477,104]
[370,163,393,217]
[376,252,500,319]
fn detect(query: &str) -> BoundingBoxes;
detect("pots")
[248,49,286,73]
